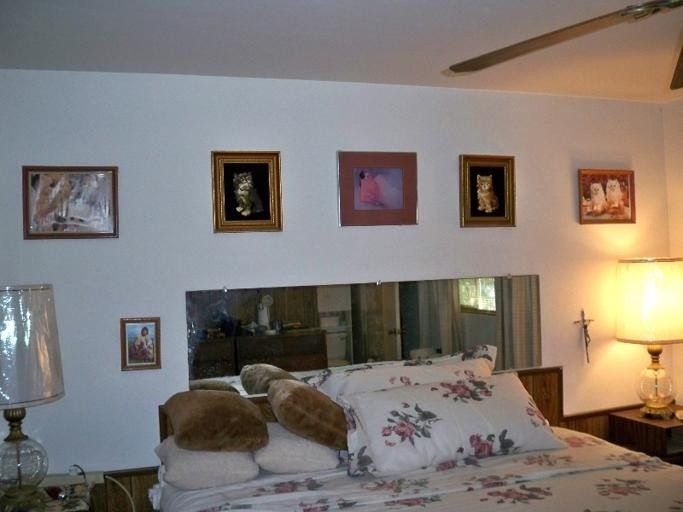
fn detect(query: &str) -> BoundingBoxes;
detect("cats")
[587,182,606,216]
[233,172,264,216]
[476,174,499,213]
[606,179,623,209]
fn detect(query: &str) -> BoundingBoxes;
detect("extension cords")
[23,470,106,485]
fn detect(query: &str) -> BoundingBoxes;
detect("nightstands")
[609,404,682,466]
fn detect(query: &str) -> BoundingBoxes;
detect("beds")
[157,366,681,512]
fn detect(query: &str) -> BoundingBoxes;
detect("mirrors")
[184,274,542,395]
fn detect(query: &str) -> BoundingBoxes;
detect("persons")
[128,325,157,364]
[359,168,386,208]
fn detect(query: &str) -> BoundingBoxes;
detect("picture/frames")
[119,317,161,371]
[458,154,515,228]
[21,165,119,240]
[576,167,635,225]
[335,149,418,227]
[210,149,283,233]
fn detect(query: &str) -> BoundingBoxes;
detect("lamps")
[612,256,682,420]
[0,283,65,511]
